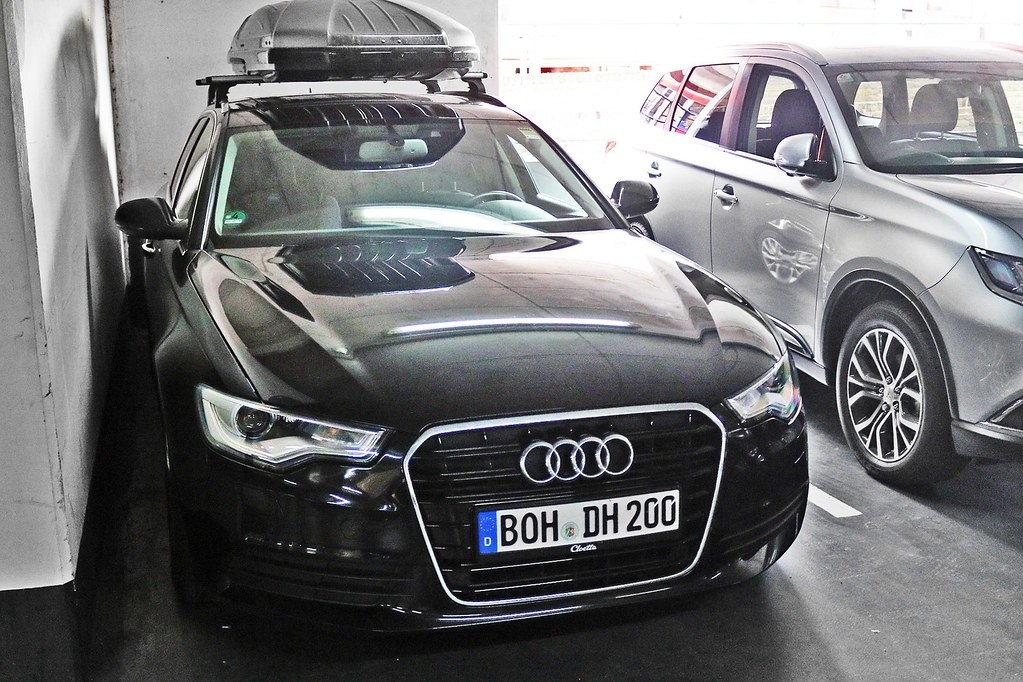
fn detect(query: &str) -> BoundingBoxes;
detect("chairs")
[415,142,478,209]
[767,89,822,157]
[236,152,344,230]
[890,82,976,155]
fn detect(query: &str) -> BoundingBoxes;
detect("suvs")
[115,0,809,637]
[599,37,1023,482]
